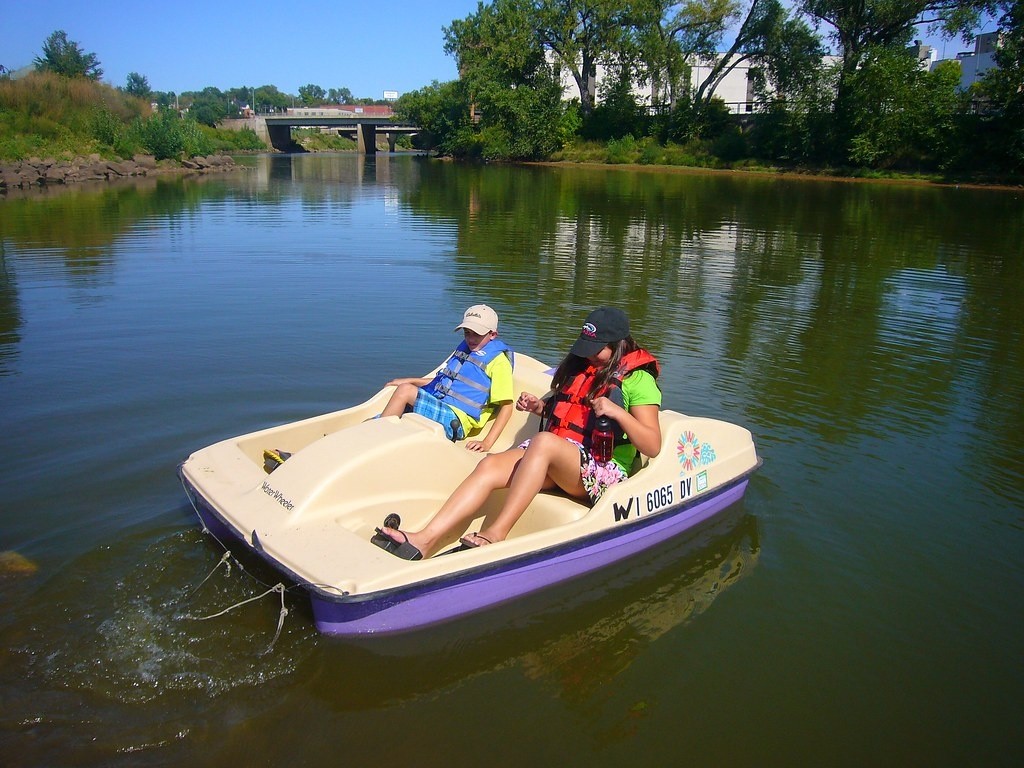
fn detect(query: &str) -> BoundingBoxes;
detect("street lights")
[972,19,993,108]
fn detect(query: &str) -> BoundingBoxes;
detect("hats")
[453,304,498,336]
[569,306,629,357]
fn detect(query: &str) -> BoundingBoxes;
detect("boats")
[181,352,764,638]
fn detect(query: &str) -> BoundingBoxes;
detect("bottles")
[592,418,614,463]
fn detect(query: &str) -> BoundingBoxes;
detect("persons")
[374,307,665,561]
[263,303,515,464]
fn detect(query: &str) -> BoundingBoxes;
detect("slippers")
[264,448,291,464]
[459,532,492,548]
[374,527,409,547]
